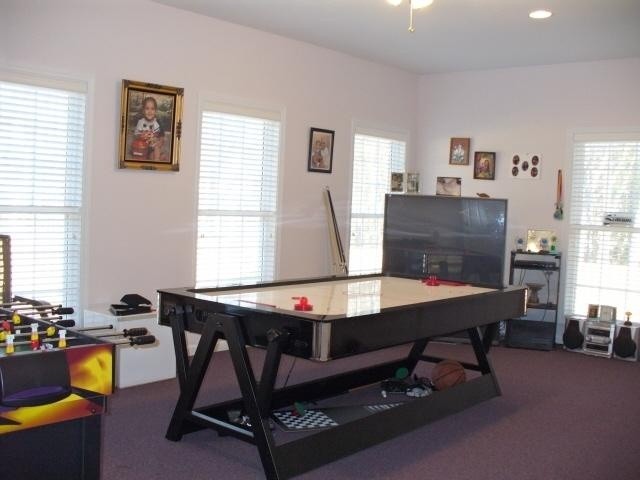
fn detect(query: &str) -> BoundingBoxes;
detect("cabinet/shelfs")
[504,248,562,351]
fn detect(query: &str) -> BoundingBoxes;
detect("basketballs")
[433,359,467,392]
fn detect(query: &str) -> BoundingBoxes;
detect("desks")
[151,273,528,480]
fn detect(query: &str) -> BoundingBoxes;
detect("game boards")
[362,402,408,414]
[270,409,340,433]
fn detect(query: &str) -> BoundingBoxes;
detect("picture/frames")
[448,136,496,181]
[119,77,183,172]
[307,126,335,173]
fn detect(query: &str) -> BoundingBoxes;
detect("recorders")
[562,313,640,362]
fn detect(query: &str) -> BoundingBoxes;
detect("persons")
[312,137,331,169]
[134,98,165,161]
[476,158,492,177]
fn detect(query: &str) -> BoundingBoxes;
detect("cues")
[322,186,349,274]
